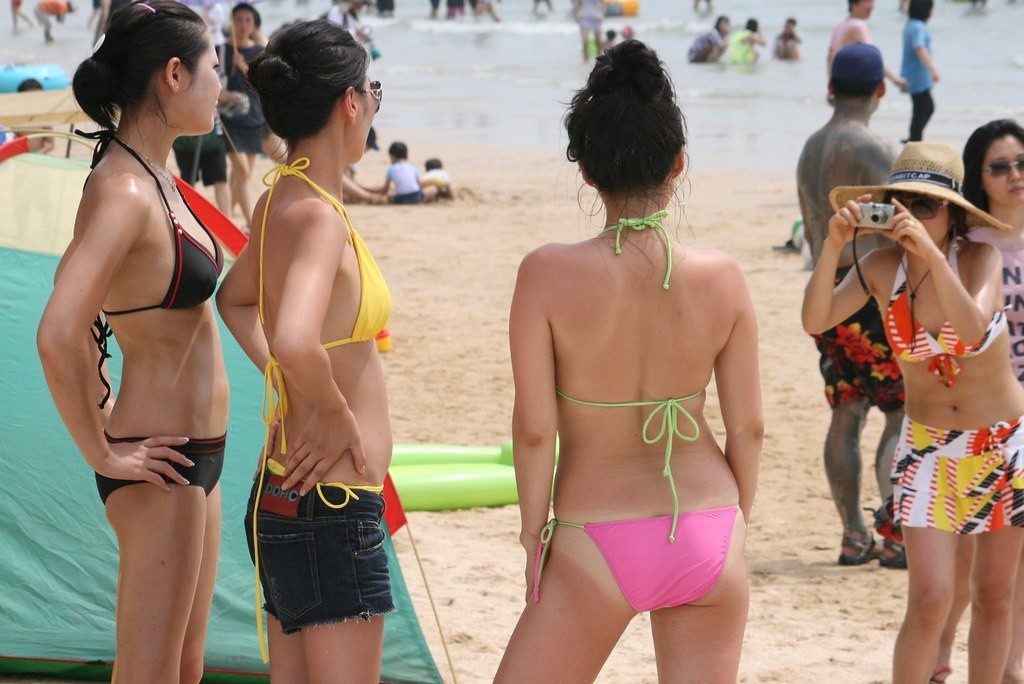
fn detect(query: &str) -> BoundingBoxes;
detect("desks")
[0,92,121,164]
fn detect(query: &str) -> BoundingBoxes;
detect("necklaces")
[119,139,176,192]
[895,236,943,352]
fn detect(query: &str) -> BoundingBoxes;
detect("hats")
[831,41,883,81]
[367,128,379,151]
[830,140,1012,233]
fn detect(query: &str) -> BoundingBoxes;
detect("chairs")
[0,132,102,258]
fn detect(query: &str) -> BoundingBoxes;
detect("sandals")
[929,668,951,684]
[878,539,907,568]
[837,535,885,565]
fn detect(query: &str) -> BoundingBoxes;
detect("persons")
[339,125,455,206]
[573,0,606,62]
[12,78,55,153]
[801,118,1024,684]
[796,42,906,571]
[321,0,395,59]
[37,0,229,684]
[493,39,763,684]
[827,0,909,105]
[687,15,732,64]
[730,19,766,65]
[774,18,802,60]
[901,0,941,143]
[430,0,501,22]
[9,0,132,47]
[215,20,400,684]
[172,0,286,237]
[603,30,619,49]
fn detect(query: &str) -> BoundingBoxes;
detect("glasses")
[342,81,383,116]
[979,155,1024,177]
[880,197,951,221]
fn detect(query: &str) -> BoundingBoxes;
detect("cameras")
[855,203,897,231]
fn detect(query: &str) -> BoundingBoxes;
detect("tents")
[0,88,457,684]
